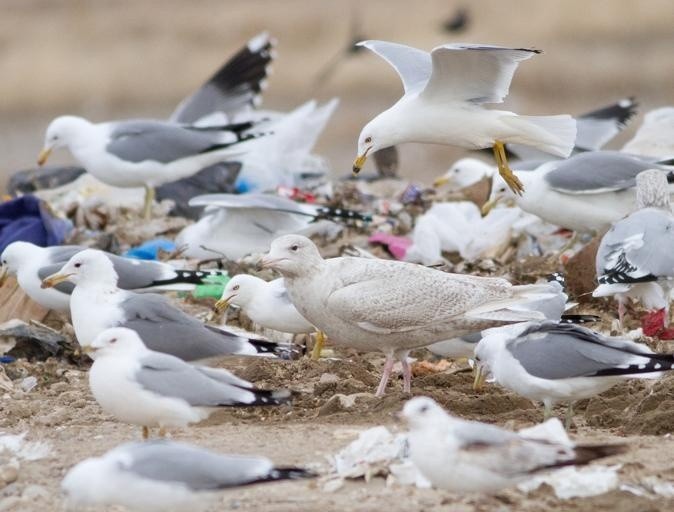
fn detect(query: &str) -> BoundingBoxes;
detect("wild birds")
[1,32,674,512]
